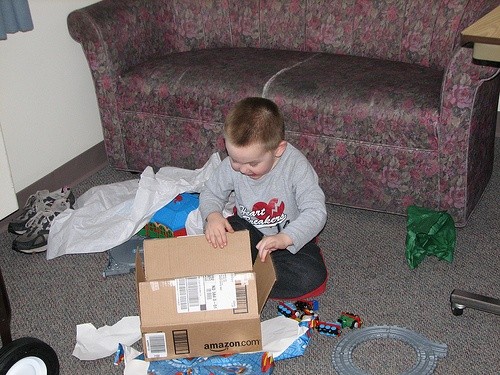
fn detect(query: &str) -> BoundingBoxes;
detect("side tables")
[460,4,500,67]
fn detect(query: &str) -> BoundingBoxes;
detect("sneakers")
[7,187,75,235]
[11,198,74,254]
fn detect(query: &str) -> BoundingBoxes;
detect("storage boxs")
[138,229,278,362]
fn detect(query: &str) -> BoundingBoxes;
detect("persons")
[199,97,328,298]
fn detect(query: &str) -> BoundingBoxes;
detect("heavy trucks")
[276,299,364,337]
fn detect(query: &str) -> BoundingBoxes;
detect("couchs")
[67,0,500,228]
